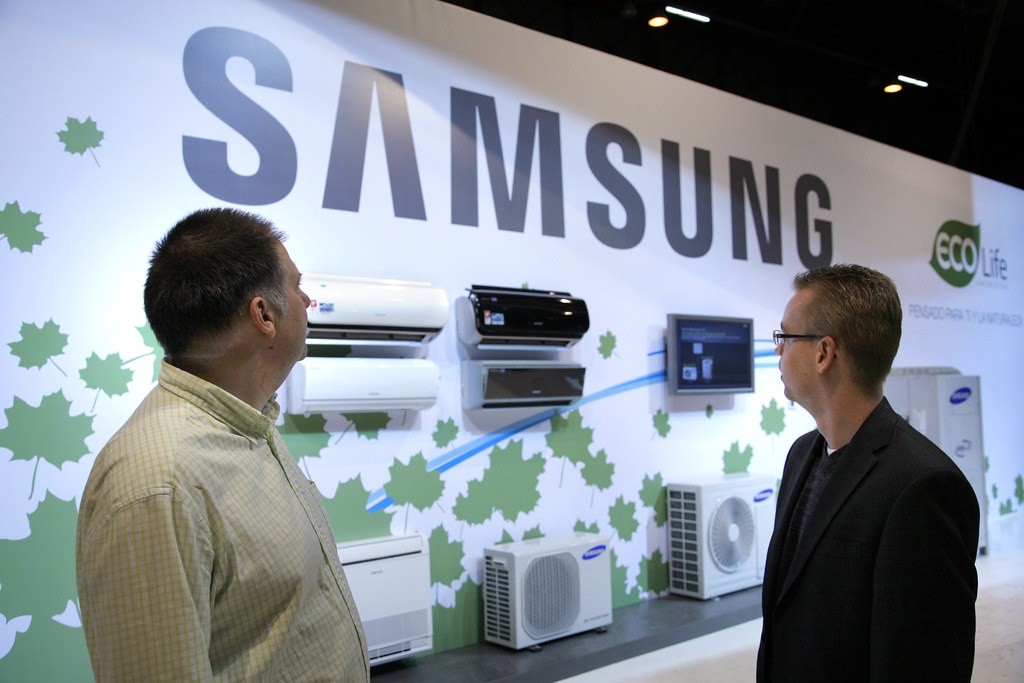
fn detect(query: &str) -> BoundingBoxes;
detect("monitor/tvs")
[667,315,755,395]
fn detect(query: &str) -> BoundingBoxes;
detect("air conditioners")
[460,359,586,411]
[331,531,433,667]
[907,376,987,556]
[483,532,613,650]
[667,474,778,600]
[455,285,589,351]
[298,272,449,347]
[286,355,440,415]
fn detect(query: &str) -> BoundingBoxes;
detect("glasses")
[772,329,827,348]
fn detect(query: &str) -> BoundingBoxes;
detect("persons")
[72,209,372,683]
[756,264,981,683]
